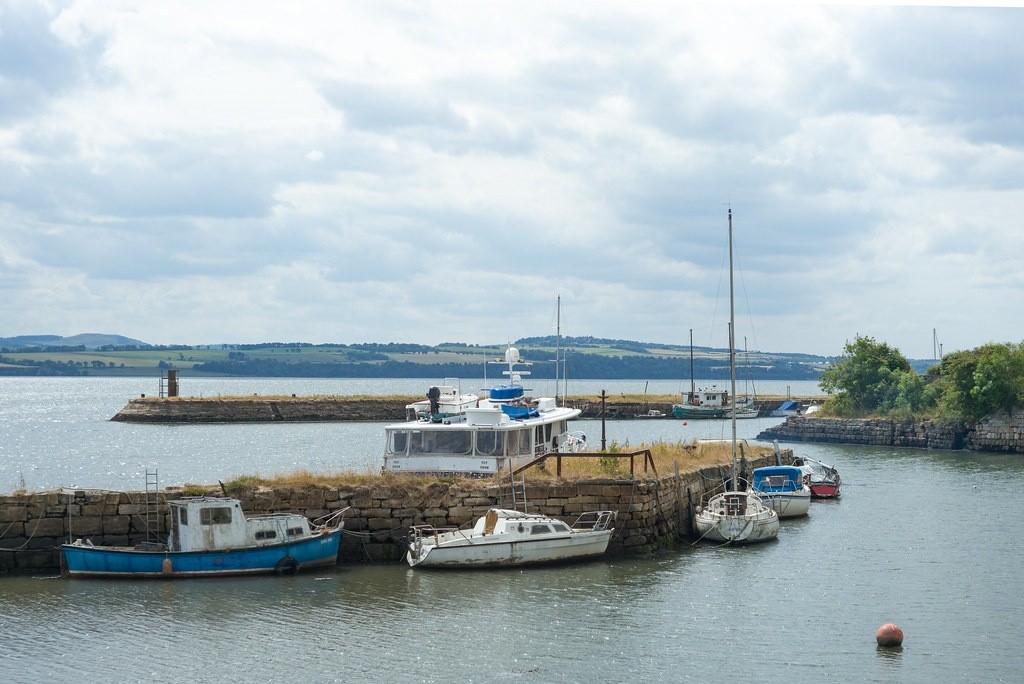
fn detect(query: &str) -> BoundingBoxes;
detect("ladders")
[146,468,159,544]
[511,473,527,515]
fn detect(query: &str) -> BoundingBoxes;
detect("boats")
[59,495,350,578]
[670,391,762,420]
[749,464,812,518]
[790,456,841,499]
[380,341,586,478]
[406,507,615,569]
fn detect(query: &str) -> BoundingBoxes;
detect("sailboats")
[693,205,780,544]
